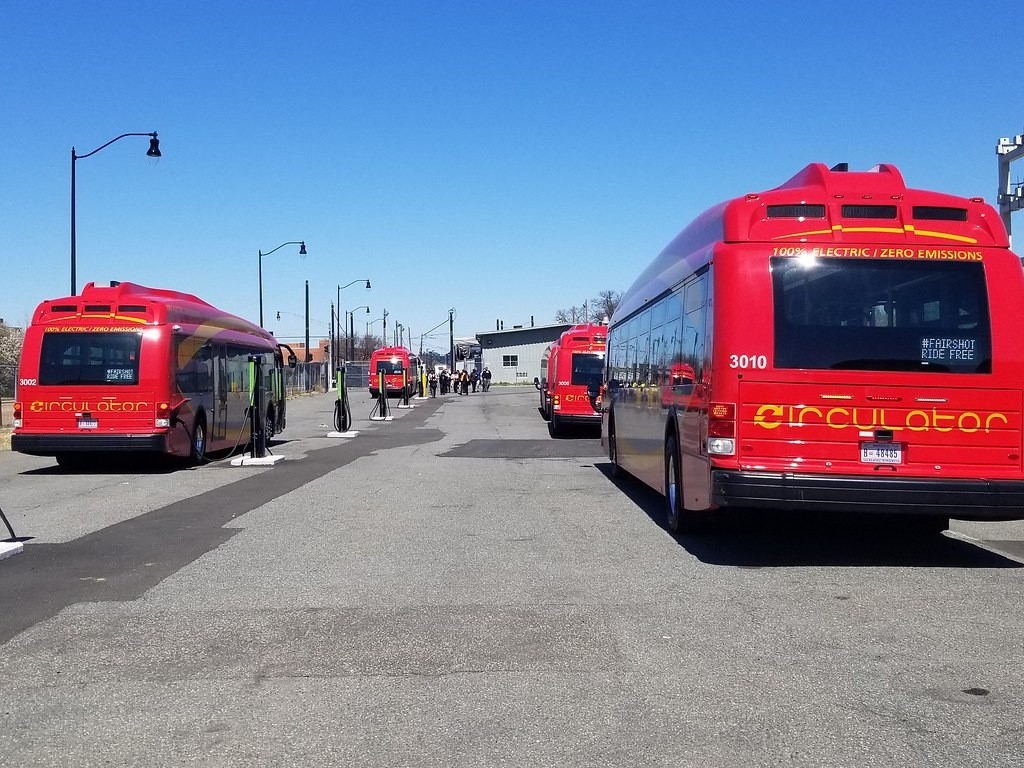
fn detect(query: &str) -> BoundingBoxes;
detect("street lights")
[276,311,334,390]
[337,278,371,386]
[345,306,370,362]
[258,240,307,328]
[70,130,161,296]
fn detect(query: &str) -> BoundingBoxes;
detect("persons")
[420,366,491,398]
[610,378,662,410]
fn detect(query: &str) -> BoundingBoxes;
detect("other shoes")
[429,391,491,398]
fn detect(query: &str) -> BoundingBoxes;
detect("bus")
[586,161,1024,539]
[11,282,297,467]
[661,363,699,411]
[368,345,421,399]
[533,322,610,433]
